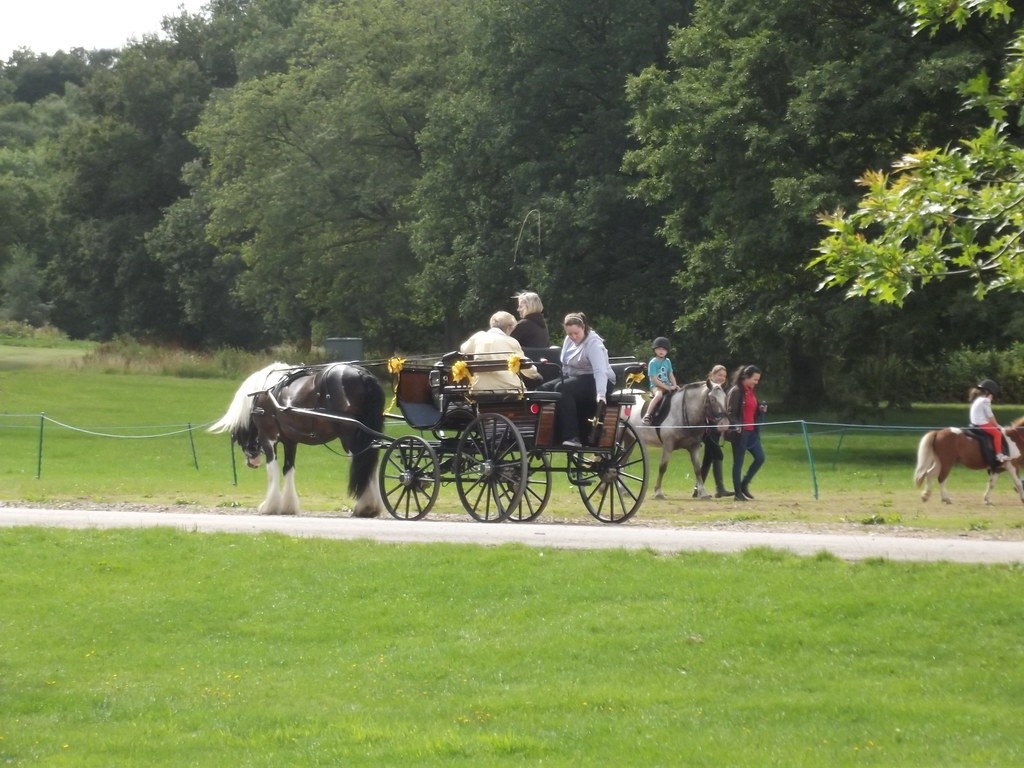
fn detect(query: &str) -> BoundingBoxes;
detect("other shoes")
[740,483,754,498]
[642,415,650,426]
[996,454,1011,461]
[692,485,699,497]
[734,488,751,501]
[715,488,735,498]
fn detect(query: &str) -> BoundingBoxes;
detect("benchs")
[442,351,564,400]
[605,356,646,404]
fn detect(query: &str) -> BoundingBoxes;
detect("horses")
[914,416,1024,504]
[208,362,385,517]
[610,378,731,499]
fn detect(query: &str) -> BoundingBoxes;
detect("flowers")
[387,356,407,411]
[508,354,525,401]
[451,360,475,406]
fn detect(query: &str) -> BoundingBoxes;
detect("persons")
[641,336,676,425]
[692,365,734,498]
[969,379,1012,461]
[509,291,550,349]
[535,312,617,447]
[727,366,767,501]
[460,311,543,394]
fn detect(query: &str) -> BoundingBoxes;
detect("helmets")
[652,337,671,353]
[977,379,999,396]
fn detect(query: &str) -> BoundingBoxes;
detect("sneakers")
[562,436,583,448]
